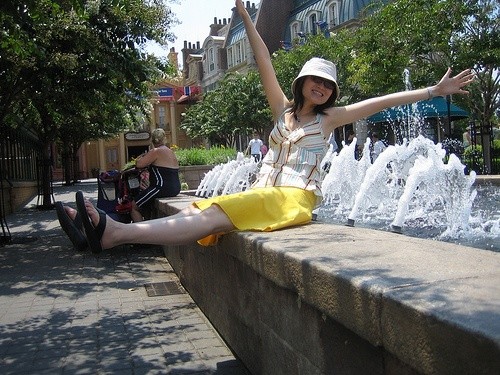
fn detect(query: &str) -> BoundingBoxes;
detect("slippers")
[56,201,88,252]
[76,191,106,255]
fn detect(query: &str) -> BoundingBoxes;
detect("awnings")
[368,95,470,122]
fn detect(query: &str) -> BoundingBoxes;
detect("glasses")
[310,76,335,90]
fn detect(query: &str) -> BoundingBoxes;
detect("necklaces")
[297,118,301,123]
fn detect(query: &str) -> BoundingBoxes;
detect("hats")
[291,57,340,100]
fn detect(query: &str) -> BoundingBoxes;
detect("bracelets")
[426,87,434,101]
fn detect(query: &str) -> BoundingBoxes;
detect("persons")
[463,128,471,145]
[56,0,477,254]
[129,128,182,223]
[244,131,268,163]
[328,130,388,164]
